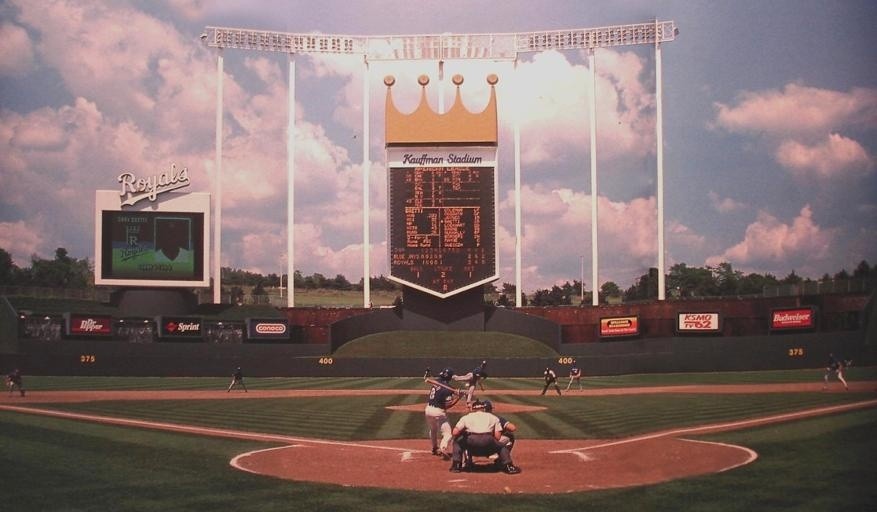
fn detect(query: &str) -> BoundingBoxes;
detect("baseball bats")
[425,379,465,393]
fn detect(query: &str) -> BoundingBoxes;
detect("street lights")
[578,254,586,301]
[278,253,286,300]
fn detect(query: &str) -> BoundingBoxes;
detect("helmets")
[471,400,484,409]
[479,361,487,368]
[483,400,495,411]
[439,368,453,379]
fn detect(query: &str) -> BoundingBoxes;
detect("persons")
[822,352,848,390]
[424,360,519,473]
[565,368,582,392]
[6,369,26,396]
[227,366,246,392]
[542,368,561,395]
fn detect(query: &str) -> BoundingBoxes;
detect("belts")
[467,432,490,436]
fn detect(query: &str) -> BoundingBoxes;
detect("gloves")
[423,366,432,377]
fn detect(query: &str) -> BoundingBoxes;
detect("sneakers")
[431,447,439,455]
[448,462,461,472]
[461,463,473,473]
[440,449,449,458]
[504,463,521,474]
